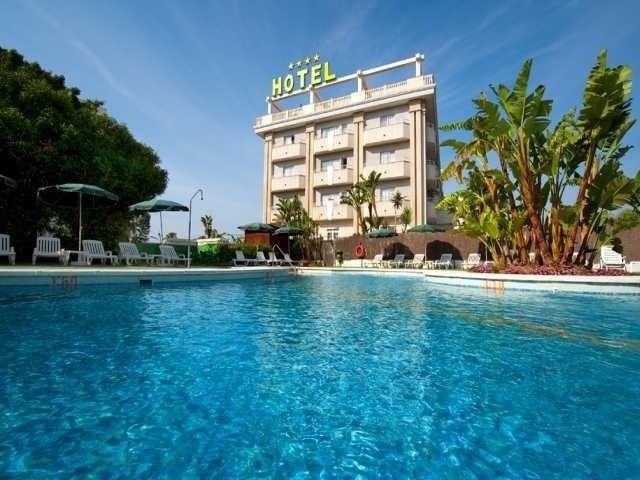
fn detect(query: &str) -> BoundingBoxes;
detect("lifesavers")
[355,245,365,257]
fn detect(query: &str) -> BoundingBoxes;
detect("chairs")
[0,234,192,267]
[231,249,299,267]
[363,253,481,272]
[599,245,627,273]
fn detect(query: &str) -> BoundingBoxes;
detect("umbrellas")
[407,223,446,262]
[237,220,275,253]
[36,179,118,250]
[129,195,189,244]
[272,222,304,260]
[364,224,398,257]
[0,173,18,189]
